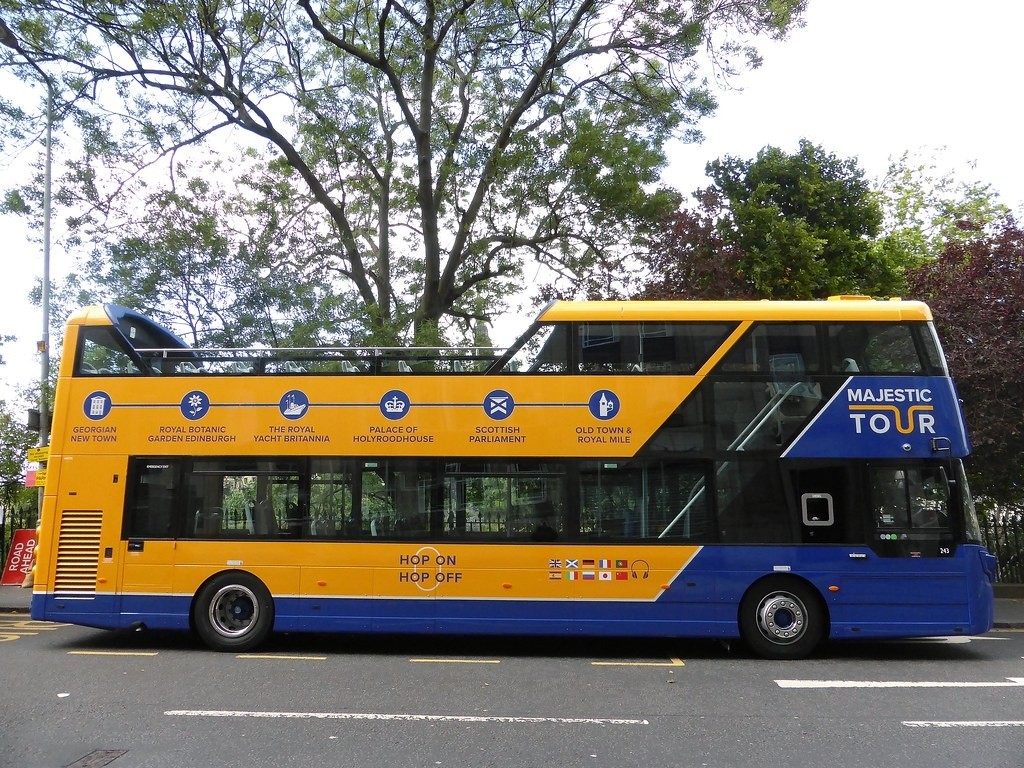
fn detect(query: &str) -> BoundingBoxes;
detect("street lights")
[0,20,55,520]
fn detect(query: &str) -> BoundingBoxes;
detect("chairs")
[191,498,640,543]
[121,355,865,376]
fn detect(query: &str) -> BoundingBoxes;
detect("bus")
[30,293,998,655]
